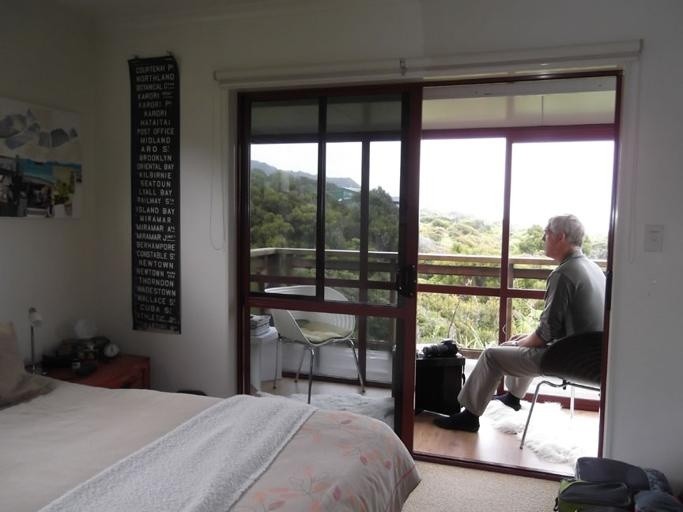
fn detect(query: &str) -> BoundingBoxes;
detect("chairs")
[519,333,606,451]
[262,284,365,405]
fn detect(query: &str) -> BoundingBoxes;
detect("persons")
[431,212,606,432]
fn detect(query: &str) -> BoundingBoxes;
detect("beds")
[0,370,421,512]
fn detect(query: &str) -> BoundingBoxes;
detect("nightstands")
[24,353,150,390]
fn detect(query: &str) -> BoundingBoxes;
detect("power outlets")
[644,224,664,252]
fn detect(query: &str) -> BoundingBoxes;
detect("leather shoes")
[433,410,479,433]
[493,392,521,411]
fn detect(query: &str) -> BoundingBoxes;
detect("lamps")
[211,58,402,84]
[403,39,646,72]
[24,305,45,372]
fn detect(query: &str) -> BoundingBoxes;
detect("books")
[249,314,271,334]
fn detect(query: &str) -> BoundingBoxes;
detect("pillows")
[0,317,59,411]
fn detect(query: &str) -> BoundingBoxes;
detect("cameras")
[423,339,459,358]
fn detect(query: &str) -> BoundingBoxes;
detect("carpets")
[484,398,599,462]
[288,391,396,421]
